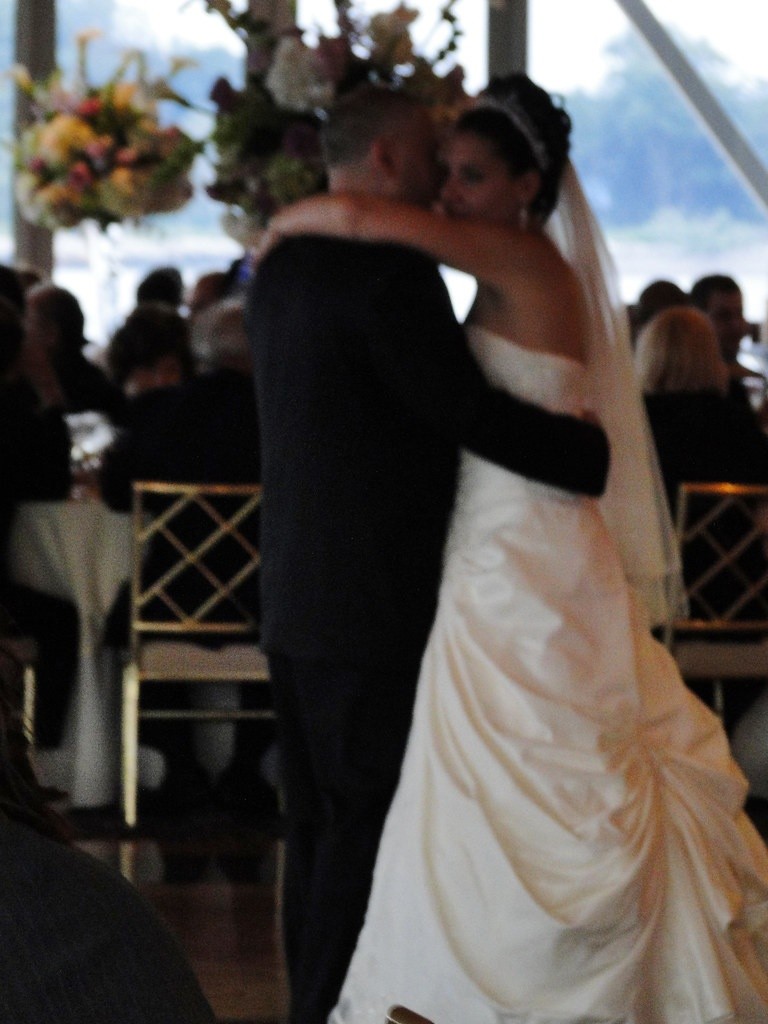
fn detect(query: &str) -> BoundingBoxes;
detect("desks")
[6,496,152,816]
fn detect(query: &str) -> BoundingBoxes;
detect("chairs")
[121,479,296,834]
[663,483,767,716]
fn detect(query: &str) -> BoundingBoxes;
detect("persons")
[0,257,286,818]
[0,646,219,1024]
[243,72,768,1024]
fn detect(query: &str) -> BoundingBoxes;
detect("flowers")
[201,0,473,236]
[1,28,208,236]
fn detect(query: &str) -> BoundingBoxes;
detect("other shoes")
[68,784,175,838]
